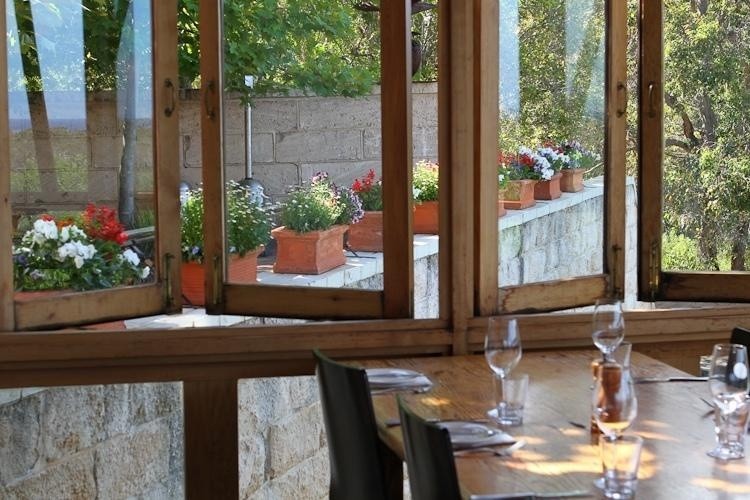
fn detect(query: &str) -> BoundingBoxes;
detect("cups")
[501,373,529,423]
[600,430,644,500]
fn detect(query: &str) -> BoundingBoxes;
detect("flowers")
[12,200,155,293]
[280,171,365,231]
[411,159,441,205]
[559,140,587,169]
[352,168,387,211]
[499,150,508,190]
[508,146,554,180]
[178,180,271,260]
[537,141,564,174]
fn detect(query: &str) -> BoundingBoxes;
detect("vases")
[411,201,440,234]
[533,174,564,200]
[346,211,385,252]
[179,246,267,306]
[499,191,507,218]
[504,181,536,209]
[272,225,351,276]
[560,168,585,192]
[13,279,163,329]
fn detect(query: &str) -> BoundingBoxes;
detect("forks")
[451,441,530,457]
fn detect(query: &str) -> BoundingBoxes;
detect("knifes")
[468,489,595,500]
[633,376,710,383]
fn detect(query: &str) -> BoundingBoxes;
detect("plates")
[434,422,501,443]
[365,368,426,385]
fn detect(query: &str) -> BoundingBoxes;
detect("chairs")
[727,326,750,390]
[397,393,461,500]
[309,346,403,500]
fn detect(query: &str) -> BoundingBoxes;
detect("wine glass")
[590,299,638,437]
[484,315,522,426]
[708,344,749,459]
